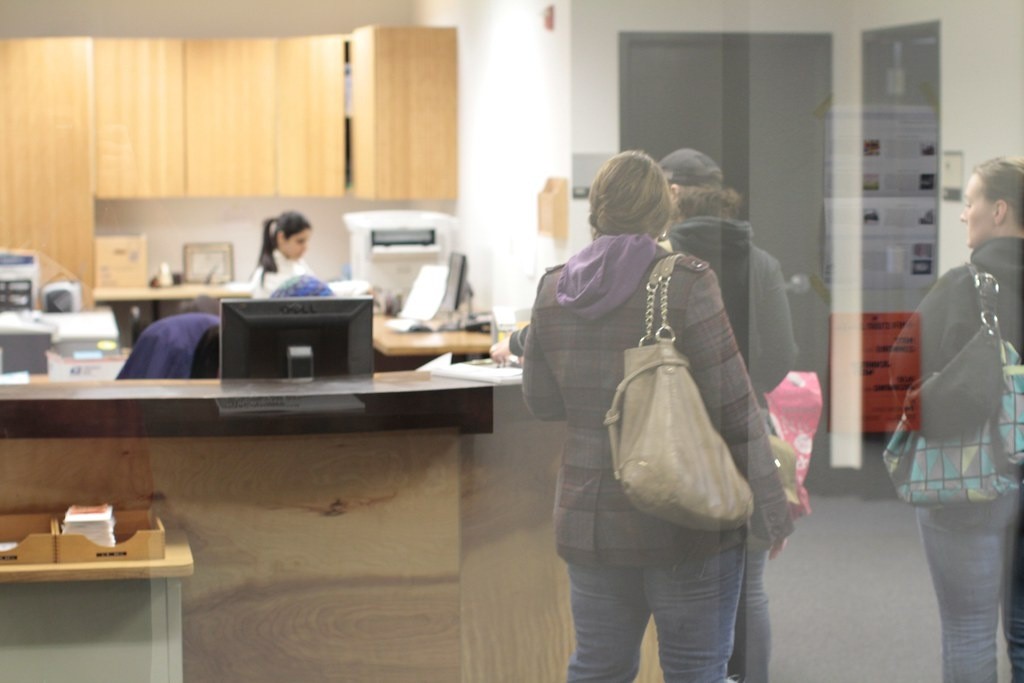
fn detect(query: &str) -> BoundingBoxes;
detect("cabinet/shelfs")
[94,36,277,201]
[278,26,460,202]
[0,36,94,312]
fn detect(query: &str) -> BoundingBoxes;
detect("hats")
[659,148,722,185]
[269,275,335,298]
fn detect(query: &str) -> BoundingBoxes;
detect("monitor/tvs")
[219,298,374,380]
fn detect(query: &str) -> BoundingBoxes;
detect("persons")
[490,147,793,683]
[522,150,794,683]
[191,213,381,381]
[886,156,1022,683]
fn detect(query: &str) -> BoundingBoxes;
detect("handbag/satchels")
[603,252,753,532]
[746,371,821,553]
[883,263,1024,506]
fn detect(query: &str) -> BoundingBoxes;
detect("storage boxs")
[54,509,164,565]
[0,512,55,564]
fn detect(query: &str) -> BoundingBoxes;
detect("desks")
[0,528,195,683]
[95,282,251,349]
[373,312,496,371]
